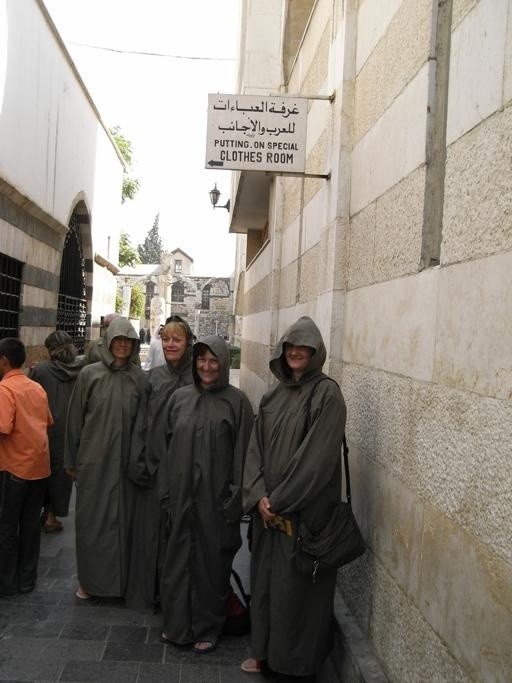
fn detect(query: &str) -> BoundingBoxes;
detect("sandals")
[44,513,64,533]
[240,656,262,673]
[191,639,217,653]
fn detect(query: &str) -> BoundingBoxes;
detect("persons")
[144,323,165,371]
[83,313,142,369]
[127,314,196,612]
[155,335,254,654]
[62,317,147,601]
[28,328,85,534]
[239,315,348,683]
[0,336,55,599]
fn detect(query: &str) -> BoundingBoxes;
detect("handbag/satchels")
[223,568,251,637]
[291,495,368,579]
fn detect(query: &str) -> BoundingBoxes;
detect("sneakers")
[1,582,36,600]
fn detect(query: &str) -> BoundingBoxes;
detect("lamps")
[208,184,233,212]
